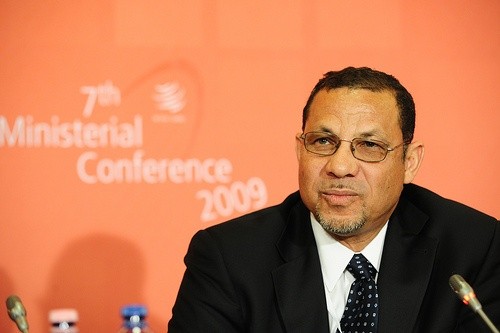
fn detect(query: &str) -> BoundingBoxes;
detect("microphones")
[449,273,500,333]
[6,295,29,333]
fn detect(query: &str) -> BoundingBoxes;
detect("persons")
[166,66,500,333]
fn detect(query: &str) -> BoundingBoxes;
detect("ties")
[336,255,380,333]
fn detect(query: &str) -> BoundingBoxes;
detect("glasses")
[299,131,407,162]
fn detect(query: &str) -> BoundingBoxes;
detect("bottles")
[47,308,80,333]
[117,304,152,333]
